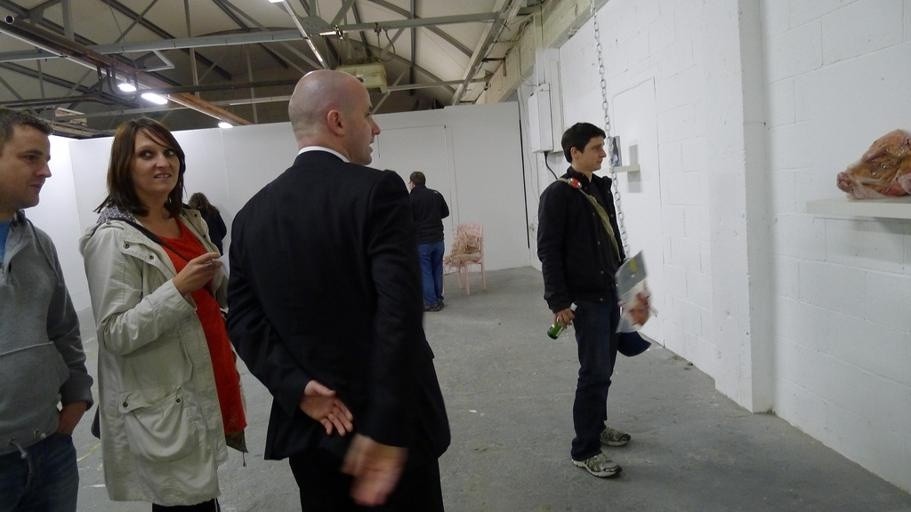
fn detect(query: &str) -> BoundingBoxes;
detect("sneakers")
[571,452,622,479]
[422,298,445,311]
[599,424,631,447]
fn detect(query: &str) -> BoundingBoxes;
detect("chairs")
[443,222,488,296]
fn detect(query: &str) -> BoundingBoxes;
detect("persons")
[186,191,228,258]
[1,104,95,512]
[408,167,450,312]
[76,114,250,511]
[536,120,631,479]
[226,68,452,510]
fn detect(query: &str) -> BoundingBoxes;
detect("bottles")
[547,301,579,340]
